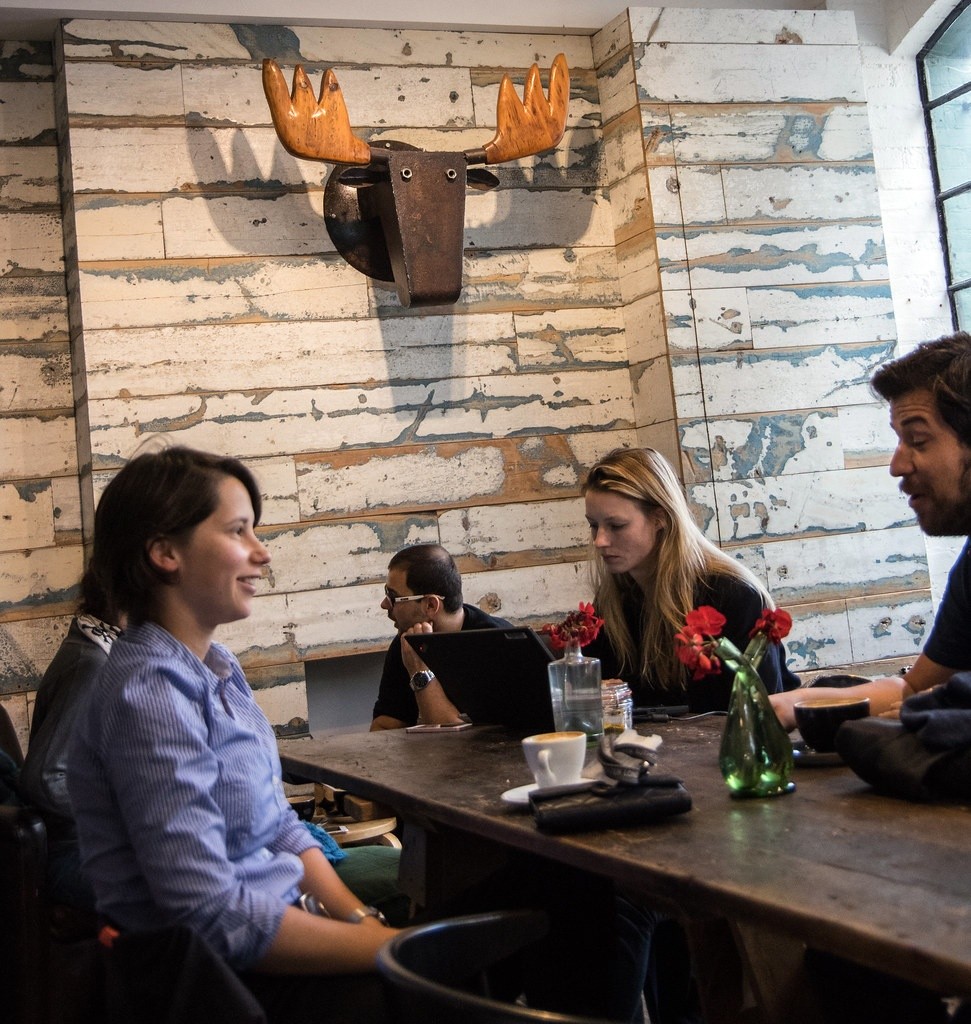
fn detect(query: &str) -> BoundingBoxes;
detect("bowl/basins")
[792,695,870,753]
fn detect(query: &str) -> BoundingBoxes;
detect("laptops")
[404,626,690,728]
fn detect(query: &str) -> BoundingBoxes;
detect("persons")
[18,549,403,917]
[370,542,514,734]
[766,329,970,1024]
[551,450,798,1024]
[63,433,414,1024]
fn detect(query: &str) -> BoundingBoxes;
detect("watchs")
[409,669,436,692]
[349,906,390,924]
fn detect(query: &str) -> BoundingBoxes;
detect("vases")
[548,640,605,747]
[717,633,796,799]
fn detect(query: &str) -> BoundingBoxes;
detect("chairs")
[380,894,644,1024]
[0,706,100,1023]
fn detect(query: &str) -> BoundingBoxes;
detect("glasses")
[385,583,445,607]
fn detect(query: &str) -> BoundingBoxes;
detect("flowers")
[539,602,605,712]
[747,606,794,644]
[675,606,740,680]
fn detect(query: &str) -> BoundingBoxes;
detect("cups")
[521,731,587,788]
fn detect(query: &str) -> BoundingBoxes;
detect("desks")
[276,693,971,1023]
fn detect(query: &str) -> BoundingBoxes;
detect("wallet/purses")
[524,770,692,833]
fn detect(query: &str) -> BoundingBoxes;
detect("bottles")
[601,679,632,734]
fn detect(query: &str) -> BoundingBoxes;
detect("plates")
[499,778,598,804]
